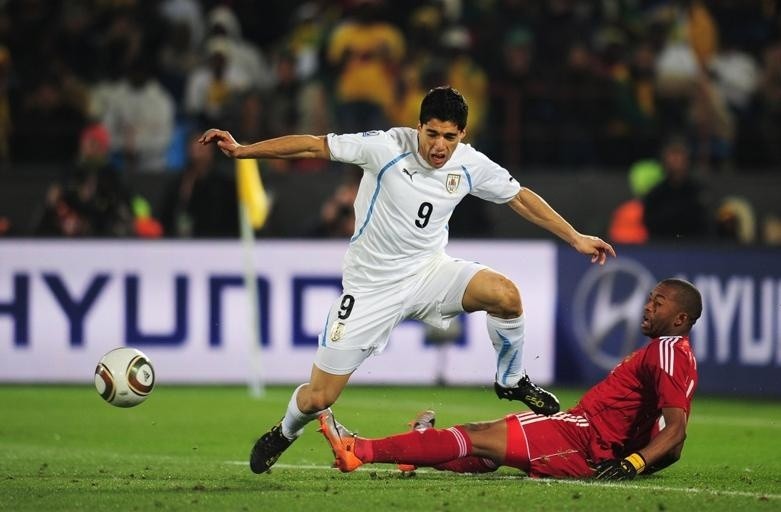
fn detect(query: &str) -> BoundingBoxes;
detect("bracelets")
[625,453,646,475]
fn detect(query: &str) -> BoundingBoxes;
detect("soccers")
[94,346,155,406]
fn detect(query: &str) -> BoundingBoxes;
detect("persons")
[317,277,702,483]
[199,86,616,475]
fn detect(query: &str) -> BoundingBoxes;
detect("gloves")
[591,451,648,482]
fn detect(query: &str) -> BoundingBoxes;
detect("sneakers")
[493,376,560,416]
[316,408,364,473]
[251,416,299,475]
[398,410,435,473]
